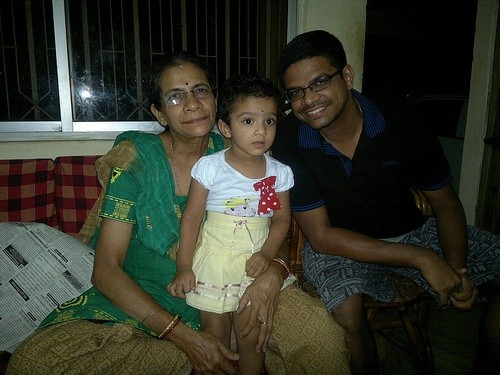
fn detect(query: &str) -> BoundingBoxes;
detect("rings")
[259,322,268,325]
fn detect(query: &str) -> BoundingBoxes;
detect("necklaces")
[163,132,206,195]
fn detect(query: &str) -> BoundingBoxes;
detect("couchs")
[0,155,106,233]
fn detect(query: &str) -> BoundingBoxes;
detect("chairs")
[289,189,437,375]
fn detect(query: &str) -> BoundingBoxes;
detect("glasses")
[283,66,342,99]
[159,81,218,105]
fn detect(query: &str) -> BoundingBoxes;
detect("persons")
[167,71,295,375]
[270,31,499,375]
[7,51,351,375]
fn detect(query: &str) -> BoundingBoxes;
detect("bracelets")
[140,308,167,324]
[158,314,182,341]
[272,256,290,280]
